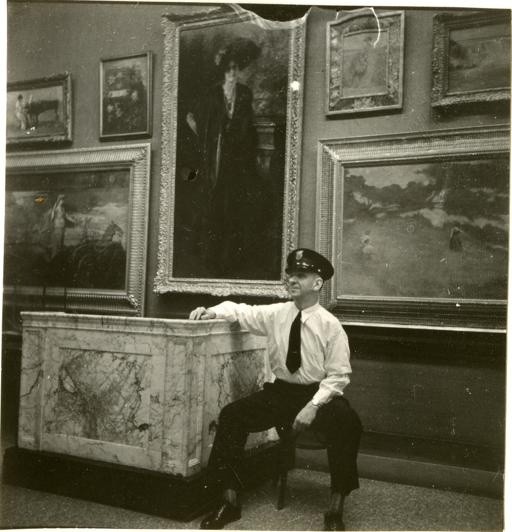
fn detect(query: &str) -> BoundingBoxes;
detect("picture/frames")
[2,143,153,316]
[313,121,510,338]
[156,7,308,304]
[7,68,76,150]
[321,10,408,117]
[98,49,158,143]
[425,11,511,117]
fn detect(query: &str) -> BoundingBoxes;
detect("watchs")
[310,398,323,409]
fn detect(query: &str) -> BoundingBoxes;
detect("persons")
[185,36,262,272]
[46,192,77,247]
[15,94,27,131]
[188,247,363,531]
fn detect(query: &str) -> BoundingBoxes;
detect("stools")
[271,423,333,510]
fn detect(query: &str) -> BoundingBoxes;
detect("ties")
[286,312,300,373]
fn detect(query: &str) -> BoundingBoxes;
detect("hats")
[285,249,333,280]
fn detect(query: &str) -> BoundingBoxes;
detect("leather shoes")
[200,501,240,529]
[324,512,344,530]
